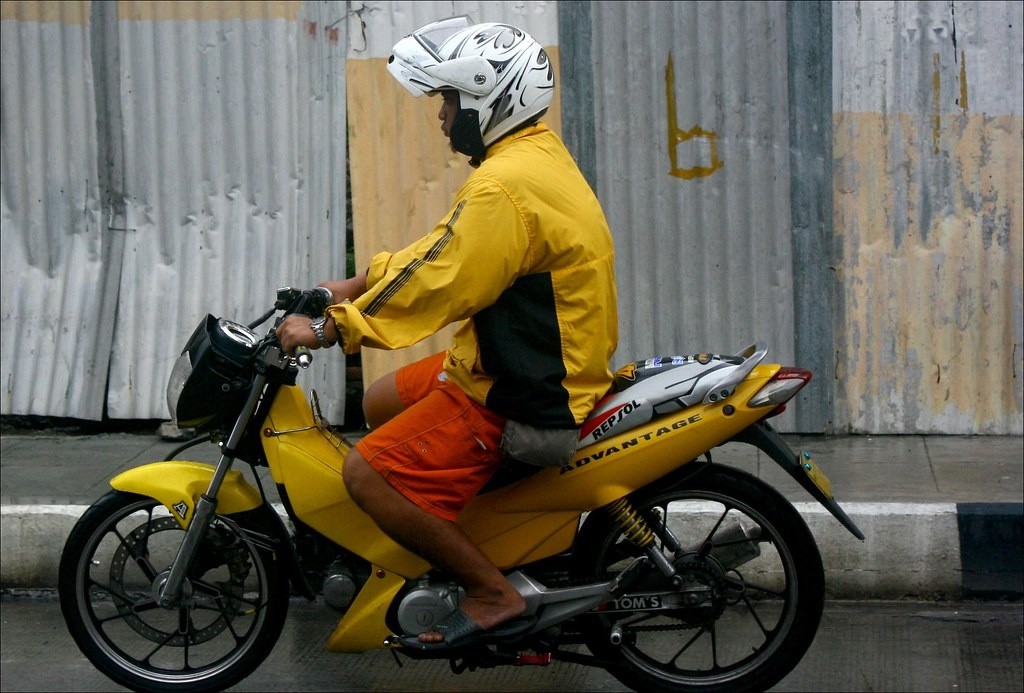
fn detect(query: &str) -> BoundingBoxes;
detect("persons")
[275,14,619,643]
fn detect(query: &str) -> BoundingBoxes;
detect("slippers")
[399,604,537,649]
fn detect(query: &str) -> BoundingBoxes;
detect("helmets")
[386,14,555,159]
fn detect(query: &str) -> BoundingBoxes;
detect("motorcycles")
[59,286,866,693]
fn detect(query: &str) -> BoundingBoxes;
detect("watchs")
[309,316,337,349]
